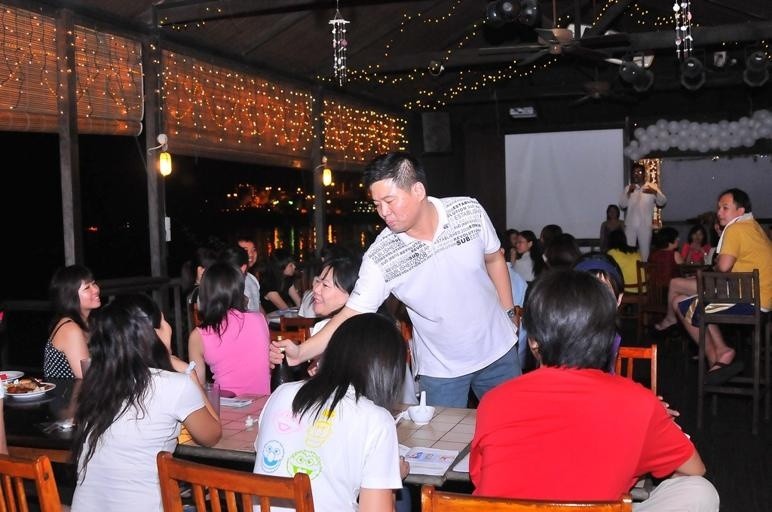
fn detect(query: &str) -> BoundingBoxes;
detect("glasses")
[632,170,645,175]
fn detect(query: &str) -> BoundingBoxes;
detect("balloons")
[624,108,772,160]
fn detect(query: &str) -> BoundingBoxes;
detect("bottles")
[266,335,294,397]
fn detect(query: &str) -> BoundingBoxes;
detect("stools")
[697,268,771,437]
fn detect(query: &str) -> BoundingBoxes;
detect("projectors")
[509,106,538,118]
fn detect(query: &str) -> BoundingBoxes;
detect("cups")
[203,382,220,418]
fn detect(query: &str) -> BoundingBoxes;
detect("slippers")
[703,360,746,387]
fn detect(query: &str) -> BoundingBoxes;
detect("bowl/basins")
[409,406,435,425]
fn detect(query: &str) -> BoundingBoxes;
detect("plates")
[1,368,61,397]
[5,397,59,408]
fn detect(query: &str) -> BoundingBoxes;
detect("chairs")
[0,260,725,511]
